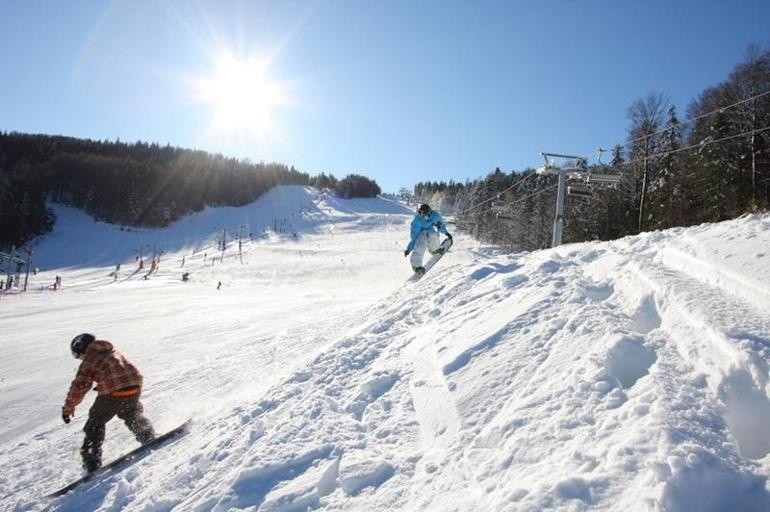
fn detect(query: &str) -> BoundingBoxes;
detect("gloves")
[62,406,74,424]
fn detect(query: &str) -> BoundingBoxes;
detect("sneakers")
[430,246,444,255]
[413,266,425,273]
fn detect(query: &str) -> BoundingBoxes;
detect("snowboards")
[404,238,452,284]
[41,420,190,499]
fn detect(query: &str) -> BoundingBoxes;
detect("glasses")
[72,350,80,359]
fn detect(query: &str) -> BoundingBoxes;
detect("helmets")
[71,333,94,354]
[417,203,432,220]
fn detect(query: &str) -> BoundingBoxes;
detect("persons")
[404,201,449,275]
[59,331,155,473]
